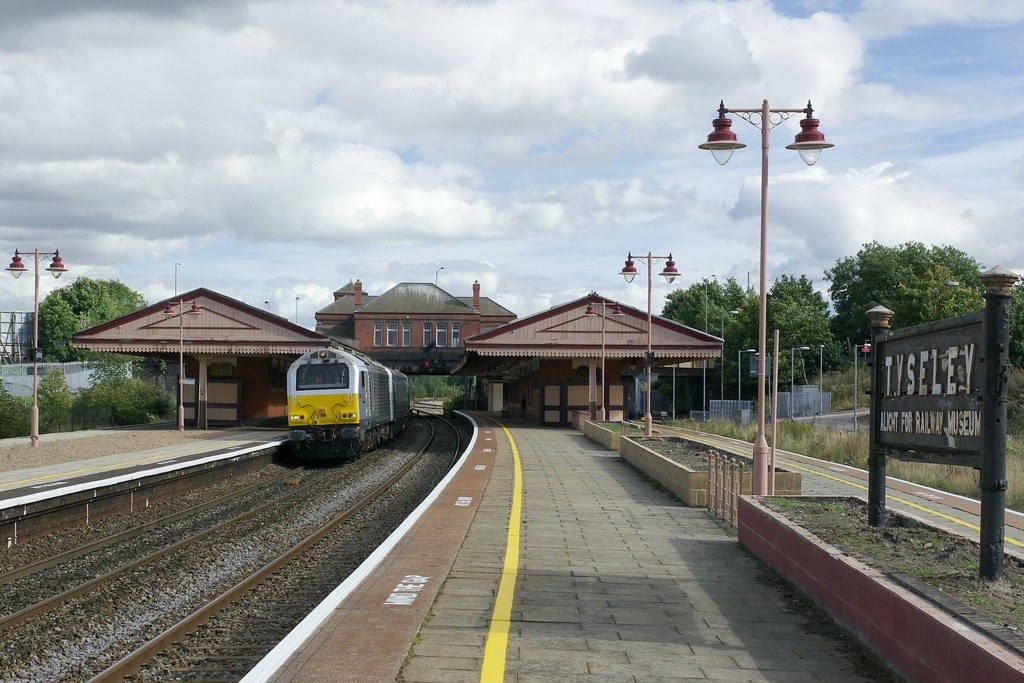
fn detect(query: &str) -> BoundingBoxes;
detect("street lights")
[160,299,203,431]
[436,266,444,286]
[738,349,756,409]
[295,296,299,325]
[5,249,68,447]
[618,252,682,436]
[697,98,835,495]
[583,299,624,421]
[791,347,810,421]
[265,301,270,311]
[721,310,739,419]
[175,262,182,296]
[705,274,716,333]
[853,344,871,432]
[820,345,825,415]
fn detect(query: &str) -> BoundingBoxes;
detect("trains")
[287,347,414,461]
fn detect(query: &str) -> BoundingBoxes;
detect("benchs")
[501,405,510,417]
